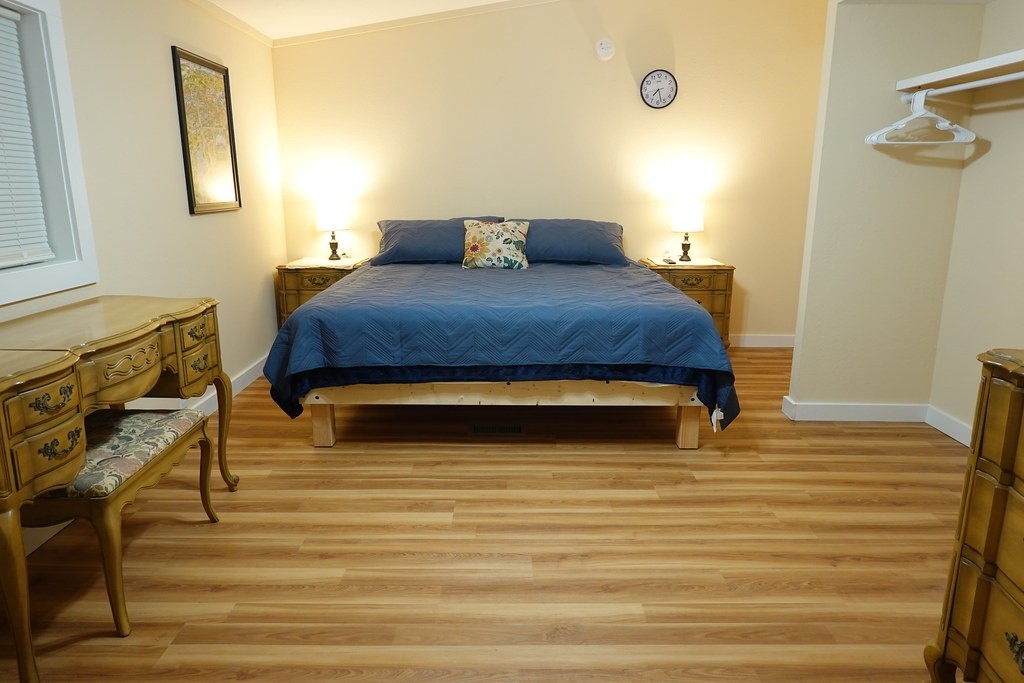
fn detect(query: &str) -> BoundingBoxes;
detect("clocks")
[640,71,677,109]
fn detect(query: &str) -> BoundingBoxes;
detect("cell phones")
[663,259,676,264]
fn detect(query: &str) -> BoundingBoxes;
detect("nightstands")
[640,258,734,349]
[274,257,367,330]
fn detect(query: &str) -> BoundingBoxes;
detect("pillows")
[524,219,627,267]
[367,216,503,266]
[462,221,530,271]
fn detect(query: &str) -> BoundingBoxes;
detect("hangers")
[864,89,977,148]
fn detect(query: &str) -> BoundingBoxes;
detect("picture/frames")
[170,46,243,216]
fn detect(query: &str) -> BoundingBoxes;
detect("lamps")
[314,196,350,260]
[673,206,705,261]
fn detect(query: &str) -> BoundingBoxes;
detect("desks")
[1,292,240,683]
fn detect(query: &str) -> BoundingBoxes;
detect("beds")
[265,217,744,456]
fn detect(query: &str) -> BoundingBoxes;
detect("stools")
[26,400,219,637]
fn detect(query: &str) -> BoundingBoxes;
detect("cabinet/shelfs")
[924,349,1024,683]
